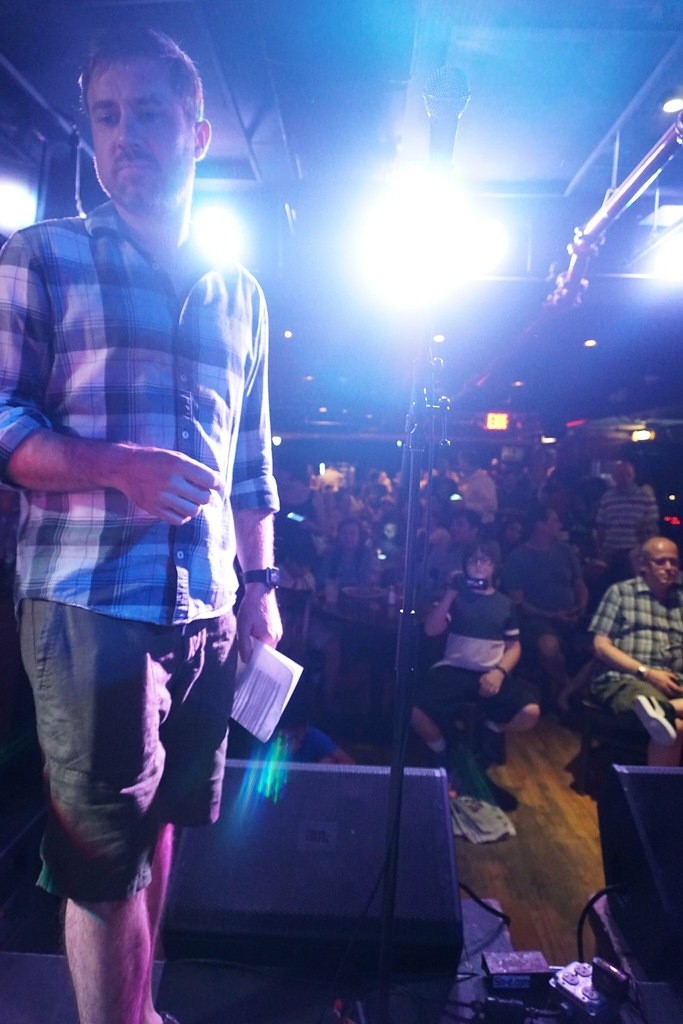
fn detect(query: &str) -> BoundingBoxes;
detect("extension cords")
[552,960,621,1022]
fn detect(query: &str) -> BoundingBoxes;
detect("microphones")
[420,63,472,165]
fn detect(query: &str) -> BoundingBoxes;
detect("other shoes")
[431,747,458,776]
[477,720,506,763]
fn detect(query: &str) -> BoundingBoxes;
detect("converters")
[483,951,552,991]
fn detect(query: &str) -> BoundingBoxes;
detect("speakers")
[595,766,682,981]
[150,757,463,979]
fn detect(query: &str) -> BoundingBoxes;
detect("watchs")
[242,567,280,591]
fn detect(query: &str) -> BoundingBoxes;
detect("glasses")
[466,555,493,566]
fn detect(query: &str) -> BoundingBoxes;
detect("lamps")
[591,763,683,985]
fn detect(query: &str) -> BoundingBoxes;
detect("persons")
[0,26,281,1024]
[234,434,683,799]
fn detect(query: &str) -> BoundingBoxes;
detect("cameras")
[451,573,488,591]
